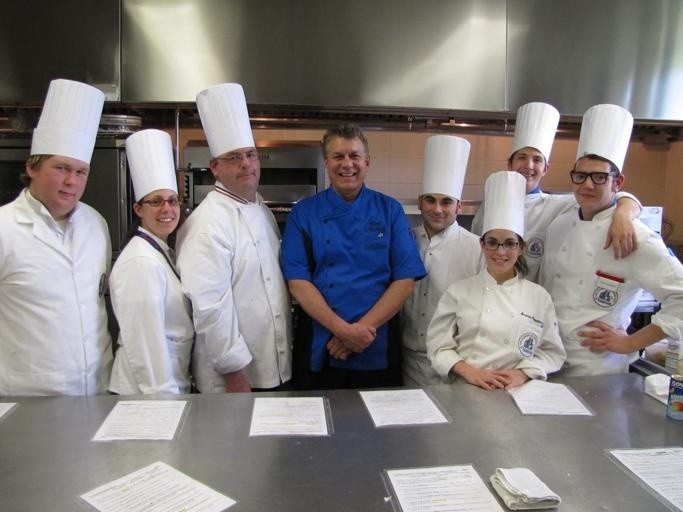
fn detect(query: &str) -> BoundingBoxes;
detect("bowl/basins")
[98,113,144,134]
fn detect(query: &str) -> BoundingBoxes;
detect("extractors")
[0,101,683,133]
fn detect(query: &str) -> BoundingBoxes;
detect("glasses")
[139,199,180,207]
[567,169,620,186]
[482,239,522,250]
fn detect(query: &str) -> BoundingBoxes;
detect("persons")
[0,79,115,397]
[279,121,428,391]
[471,102,642,283]
[387,135,487,387]
[536,103,683,375]
[108,128,195,394]
[175,81,295,392]
[426,170,567,393]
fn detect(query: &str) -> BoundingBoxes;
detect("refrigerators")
[0,137,138,266]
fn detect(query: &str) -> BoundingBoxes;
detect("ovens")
[185,141,327,239]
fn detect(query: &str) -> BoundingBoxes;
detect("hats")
[479,171,526,245]
[124,128,179,204]
[195,83,255,160]
[574,103,634,176]
[418,134,470,203]
[508,102,561,168]
[29,79,105,165]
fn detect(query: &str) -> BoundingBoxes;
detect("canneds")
[667,375,683,421]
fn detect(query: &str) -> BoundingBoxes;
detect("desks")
[1,374,682,511]
